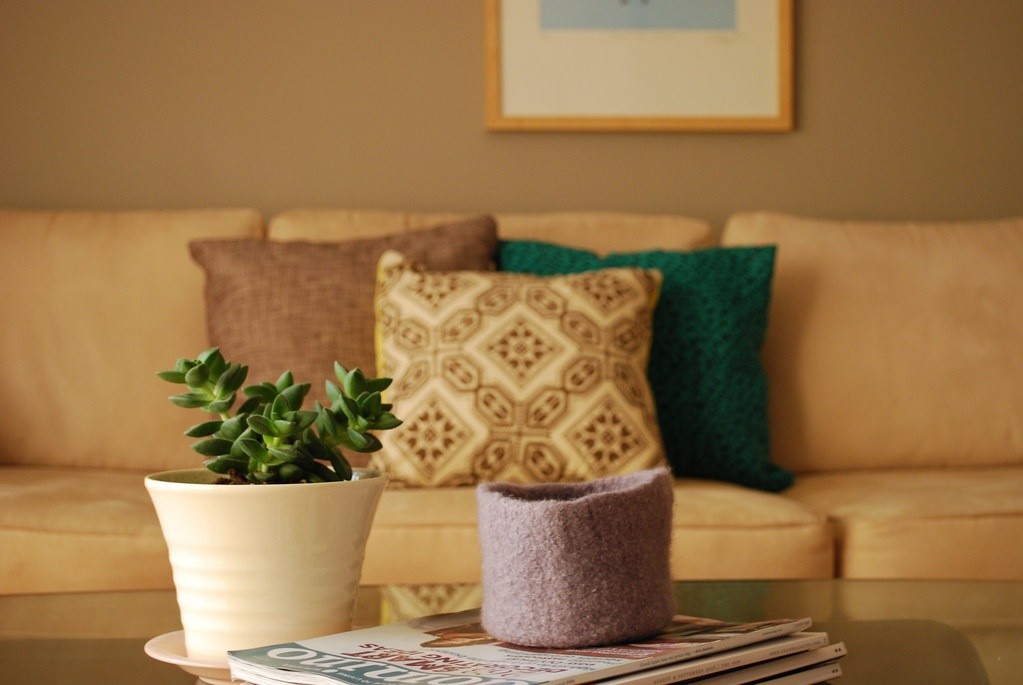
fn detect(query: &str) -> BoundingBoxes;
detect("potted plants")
[143,347,403,660]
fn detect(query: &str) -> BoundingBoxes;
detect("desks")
[0,616,992,685]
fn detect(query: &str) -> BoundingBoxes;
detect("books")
[226,608,848,685]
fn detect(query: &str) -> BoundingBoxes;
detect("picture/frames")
[481,0,798,135]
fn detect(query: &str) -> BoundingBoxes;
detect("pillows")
[367,250,673,488]
[187,214,496,468]
[496,239,797,494]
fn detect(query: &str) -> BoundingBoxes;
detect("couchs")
[0,207,1023,579]
